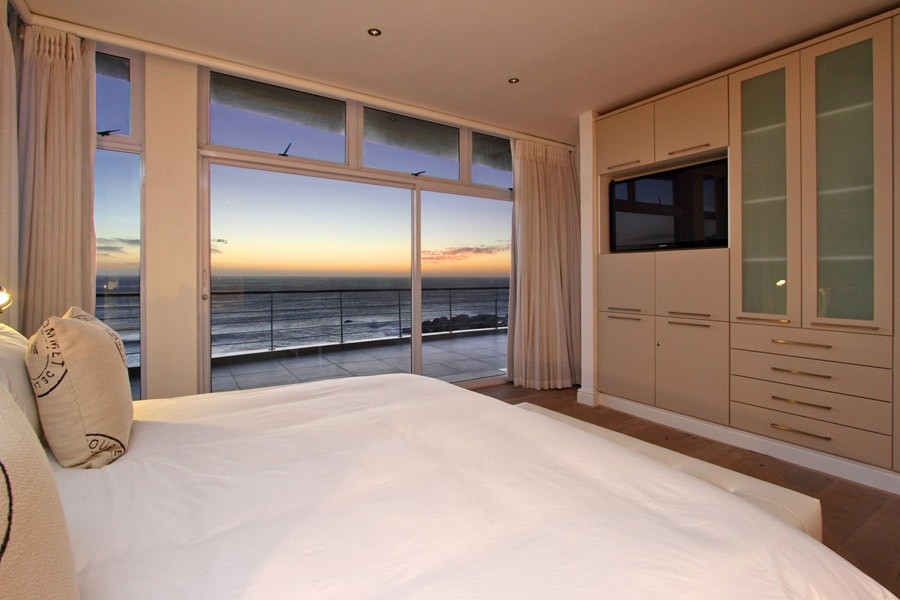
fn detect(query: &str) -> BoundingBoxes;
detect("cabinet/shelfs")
[597,8,900,473]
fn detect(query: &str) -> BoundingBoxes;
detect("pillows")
[0,384,81,600]
[24,305,134,469]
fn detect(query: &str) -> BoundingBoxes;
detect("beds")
[0,322,898,600]
